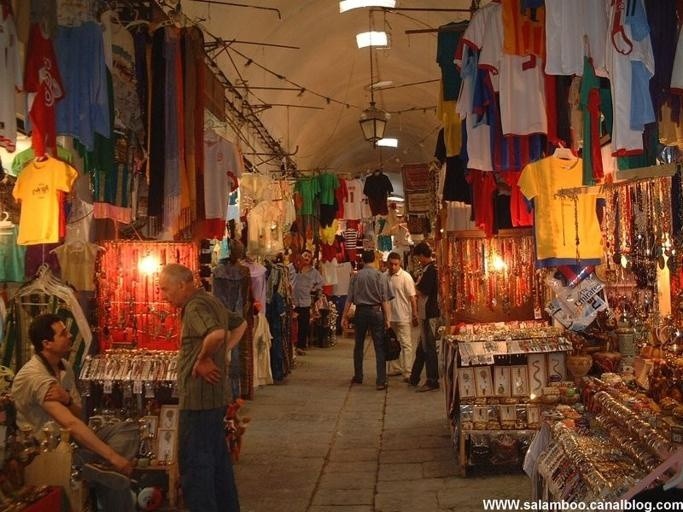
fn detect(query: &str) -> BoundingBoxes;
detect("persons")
[157,263,247,512]
[287,249,322,355]
[341,250,394,390]
[9,312,142,511]
[403,241,441,393]
[382,252,419,384]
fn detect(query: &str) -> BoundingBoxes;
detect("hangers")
[102,4,201,36]
[549,137,574,164]
[14,262,70,307]
[0,211,19,238]
[26,150,56,168]
[204,115,220,142]
[49,226,108,256]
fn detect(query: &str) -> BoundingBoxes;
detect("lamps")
[358,9,386,152]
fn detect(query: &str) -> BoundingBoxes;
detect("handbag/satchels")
[385,327,400,361]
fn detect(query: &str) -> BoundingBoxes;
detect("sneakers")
[81,462,132,492]
[377,371,437,391]
[352,376,362,383]
